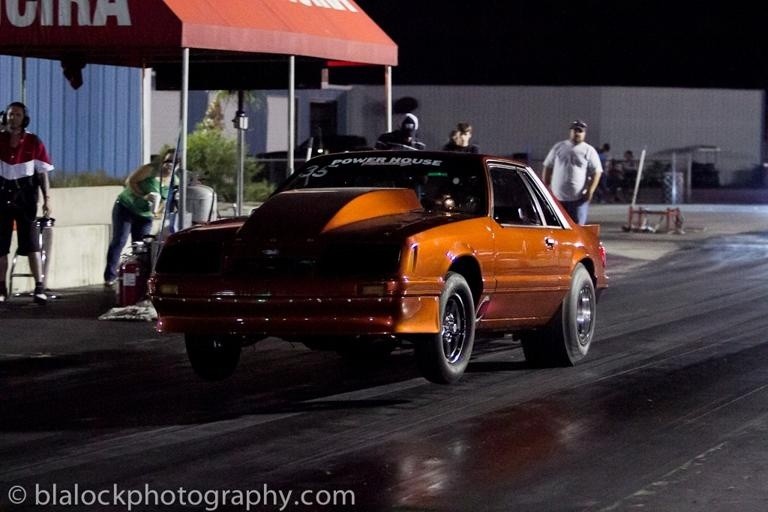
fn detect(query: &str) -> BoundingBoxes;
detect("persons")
[375,114,427,151]
[610,160,632,202]
[623,148,643,204]
[542,120,604,224]
[0,102,56,305]
[104,148,179,285]
[595,140,612,203]
[442,123,480,152]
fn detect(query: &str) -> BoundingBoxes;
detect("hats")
[401,116,415,138]
[569,119,587,132]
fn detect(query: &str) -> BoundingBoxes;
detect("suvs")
[253,132,376,161]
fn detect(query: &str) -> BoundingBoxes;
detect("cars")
[147,146,608,382]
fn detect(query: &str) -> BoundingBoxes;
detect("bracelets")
[44,195,51,199]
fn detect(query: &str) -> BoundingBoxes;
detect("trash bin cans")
[663,171,685,205]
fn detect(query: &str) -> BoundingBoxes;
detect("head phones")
[0,101,30,128]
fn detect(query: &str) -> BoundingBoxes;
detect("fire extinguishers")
[119,234,158,308]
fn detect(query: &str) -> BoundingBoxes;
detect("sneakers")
[106,276,119,286]
[0,289,8,302]
[34,288,56,300]
